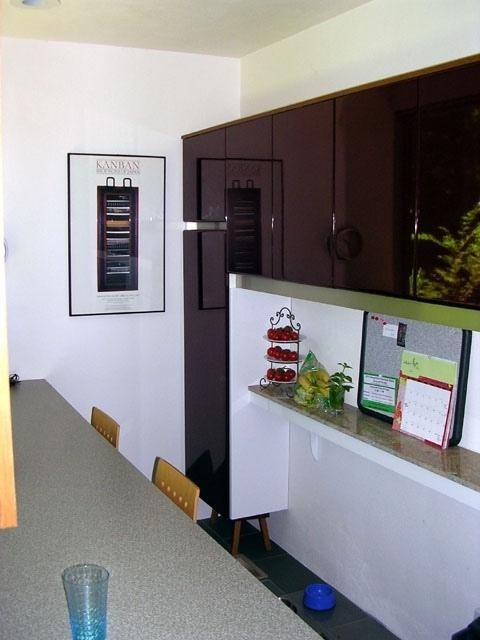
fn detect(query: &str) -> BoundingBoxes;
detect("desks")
[1,380,326,640]
[248,375,480,639]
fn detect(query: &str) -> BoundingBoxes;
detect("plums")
[267,346,298,361]
[266,366,296,383]
[267,326,298,341]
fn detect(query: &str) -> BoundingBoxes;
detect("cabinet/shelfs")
[181,128,289,522]
[271,93,334,309]
[260,307,303,398]
[416,53,479,331]
[225,110,273,303]
[333,72,416,322]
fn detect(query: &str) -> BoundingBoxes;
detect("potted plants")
[324,362,354,412]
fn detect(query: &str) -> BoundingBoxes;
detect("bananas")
[294,367,331,408]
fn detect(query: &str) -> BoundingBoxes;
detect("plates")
[264,375,297,384]
[263,333,305,343]
[265,354,305,364]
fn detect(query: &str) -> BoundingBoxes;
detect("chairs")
[152,458,199,523]
[89,406,118,448]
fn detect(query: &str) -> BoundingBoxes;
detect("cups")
[62,563,109,640]
